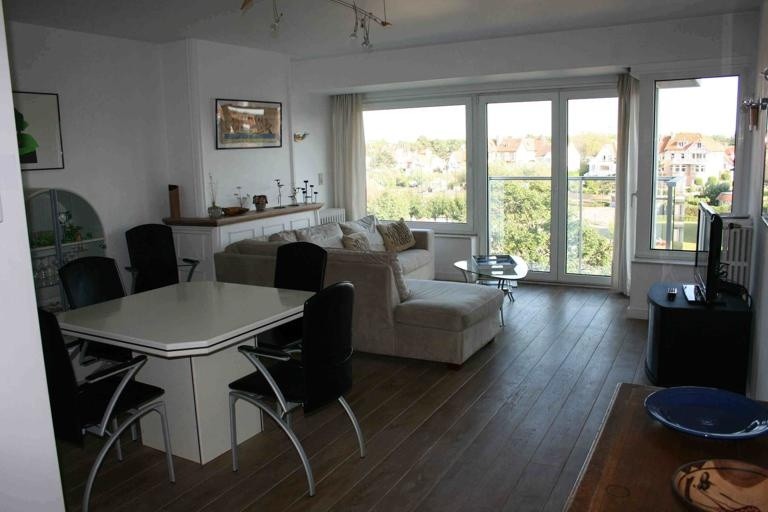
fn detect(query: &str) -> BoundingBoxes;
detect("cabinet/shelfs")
[25,187,106,313]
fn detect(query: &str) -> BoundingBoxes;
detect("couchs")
[214,215,504,368]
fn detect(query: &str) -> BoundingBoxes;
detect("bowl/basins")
[221,206,250,216]
[643,384,768,444]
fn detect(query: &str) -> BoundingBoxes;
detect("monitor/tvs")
[682,202,723,303]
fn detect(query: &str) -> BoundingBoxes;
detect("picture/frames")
[11,90,65,171]
[215,98,282,149]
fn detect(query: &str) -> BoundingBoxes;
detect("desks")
[562,382,768,512]
[644,283,753,397]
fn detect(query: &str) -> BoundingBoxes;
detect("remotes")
[667,287,678,294]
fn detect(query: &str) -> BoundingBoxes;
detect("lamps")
[263,0,394,52]
[742,96,768,131]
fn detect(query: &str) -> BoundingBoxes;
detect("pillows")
[236,215,416,302]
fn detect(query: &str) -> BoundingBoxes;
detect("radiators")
[728,222,753,291]
